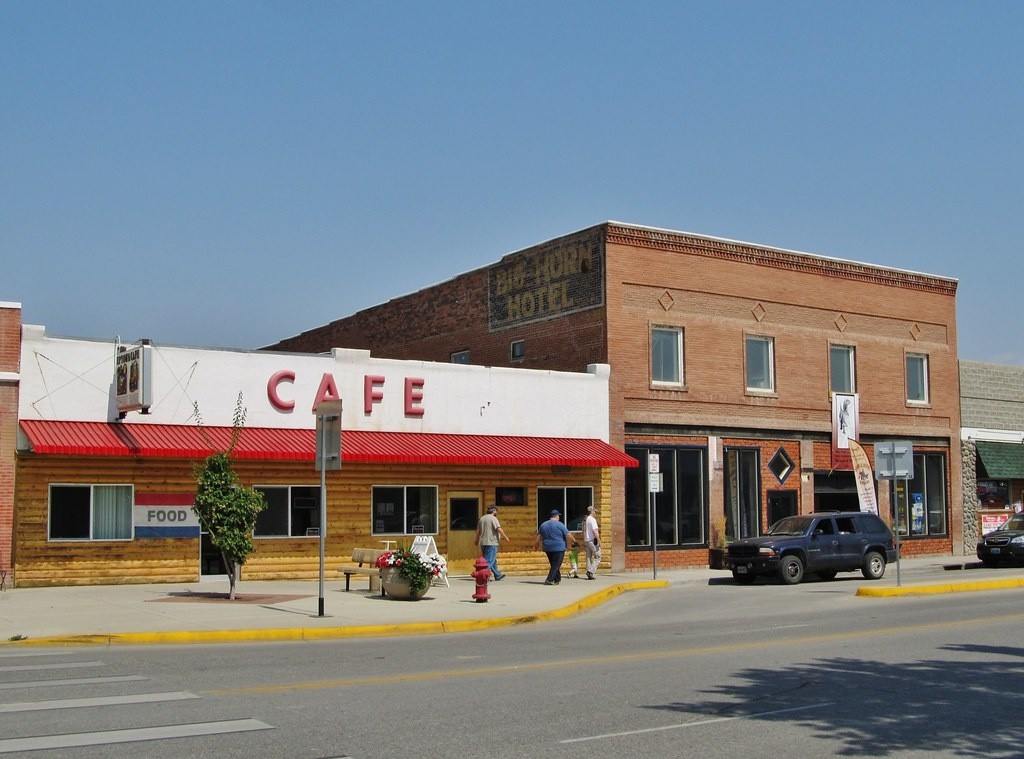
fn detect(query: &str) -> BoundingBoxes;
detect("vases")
[380,567,431,599]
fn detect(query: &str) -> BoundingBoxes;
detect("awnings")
[976,442,1024,479]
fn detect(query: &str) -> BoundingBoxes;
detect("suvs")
[723,510,897,586]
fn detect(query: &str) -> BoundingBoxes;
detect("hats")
[550,510,562,516]
[571,543,580,548]
[487,506,495,511]
[587,506,596,511]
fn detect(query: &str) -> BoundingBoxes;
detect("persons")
[566,542,583,579]
[583,506,602,580]
[474,504,509,581]
[535,510,575,585]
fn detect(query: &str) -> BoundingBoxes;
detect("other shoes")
[495,574,505,580]
[586,572,596,579]
[574,576,578,578]
[545,580,552,585]
[566,572,571,578]
[555,581,559,584]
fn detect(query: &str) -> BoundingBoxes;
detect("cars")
[976,509,1024,568]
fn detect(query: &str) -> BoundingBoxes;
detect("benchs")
[337,548,399,592]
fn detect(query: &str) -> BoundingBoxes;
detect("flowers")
[375,539,446,599]
